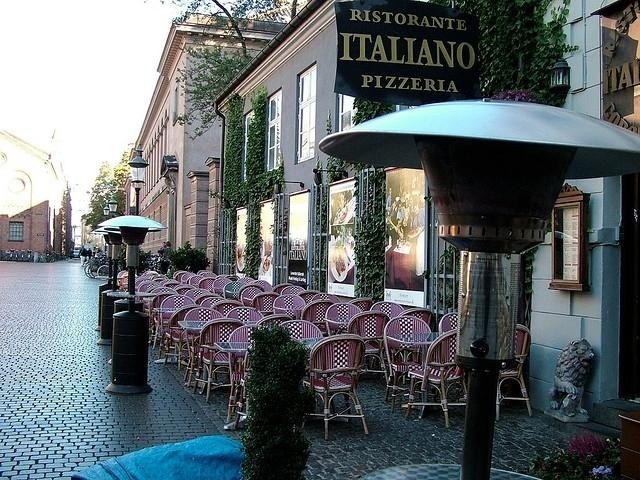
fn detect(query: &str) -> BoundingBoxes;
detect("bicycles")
[83,253,171,281]
[0,247,61,263]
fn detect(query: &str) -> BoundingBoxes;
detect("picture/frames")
[547,182,592,292]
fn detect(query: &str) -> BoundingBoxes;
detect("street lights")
[103,202,111,257]
[127,147,148,276]
[110,194,118,217]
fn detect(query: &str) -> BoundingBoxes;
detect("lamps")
[548,58,571,107]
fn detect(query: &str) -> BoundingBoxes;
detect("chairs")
[495,323,533,422]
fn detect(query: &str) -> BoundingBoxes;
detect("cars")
[73,250,81,258]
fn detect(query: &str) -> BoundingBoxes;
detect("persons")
[383,191,426,287]
[78,245,88,266]
[158,241,173,274]
[93,246,96,257]
[68,247,74,259]
[158,242,166,264]
[88,248,93,259]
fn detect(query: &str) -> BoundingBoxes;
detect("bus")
[74,246,82,250]
